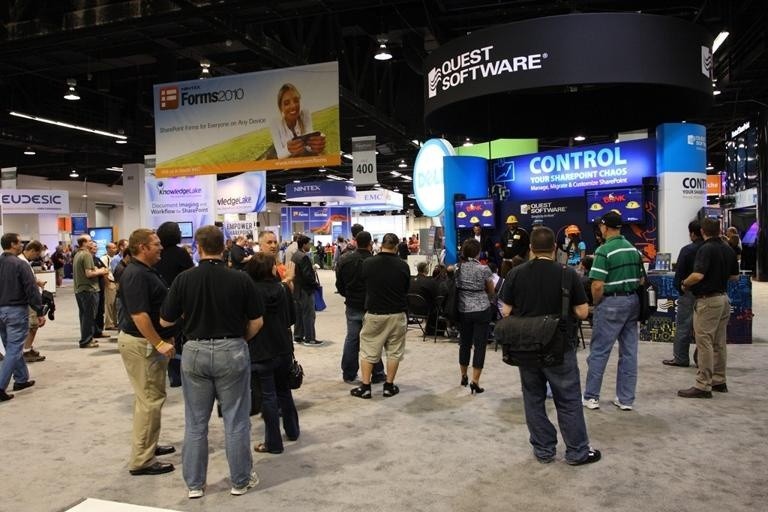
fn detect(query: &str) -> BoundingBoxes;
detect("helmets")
[568,224,580,235]
[505,214,518,223]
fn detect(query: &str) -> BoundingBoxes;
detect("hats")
[595,212,622,228]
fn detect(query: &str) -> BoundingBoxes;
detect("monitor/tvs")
[86,226,114,259]
[584,188,645,223]
[699,207,724,223]
[174,222,193,239]
[455,199,497,231]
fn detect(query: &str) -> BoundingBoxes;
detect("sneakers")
[293,337,305,343]
[372,374,386,384]
[304,340,324,346]
[612,398,633,411]
[342,374,360,385]
[231,471,259,495]
[351,387,371,398]
[566,448,601,466]
[383,386,399,396]
[582,396,600,409]
[188,488,204,498]
[537,453,553,462]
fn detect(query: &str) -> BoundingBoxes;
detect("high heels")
[461,375,468,387]
[470,382,484,394]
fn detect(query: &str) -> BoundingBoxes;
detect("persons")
[225,239,233,266]
[471,222,496,260]
[343,224,363,255]
[57,241,78,264]
[100,242,119,330]
[581,210,646,410]
[244,230,282,282]
[111,239,128,271]
[407,262,439,336]
[726,226,742,248]
[245,251,301,455]
[408,234,419,254]
[73,234,108,348]
[372,236,381,255]
[230,234,256,269]
[486,263,507,320]
[245,239,255,256]
[283,233,301,265]
[336,231,388,385]
[316,240,324,270]
[399,235,410,259]
[723,235,741,268]
[495,215,529,277]
[433,262,459,328]
[529,217,556,261]
[272,84,326,158]
[90,240,111,337]
[1,233,41,401]
[113,247,131,333]
[456,240,496,396]
[116,228,176,476]
[678,217,729,398]
[351,233,411,400]
[564,224,588,278]
[497,227,600,466]
[332,234,350,293]
[52,247,65,287]
[291,235,325,347]
[662,221,706,368]
[151,222,197,388]
[15,239,46,361]
[160,223,264,498]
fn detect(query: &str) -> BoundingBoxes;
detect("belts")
[121,328,143,338]
[603,291,634,296]
[696,291,726,297]
[190,334,241,340]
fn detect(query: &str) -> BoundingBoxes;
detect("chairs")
[489,320,501,351]
[577,278,593,350]
[434,296,452,342]
[405,293,431,341]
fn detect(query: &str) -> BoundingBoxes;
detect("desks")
[646,269,753,275]
[640,275,753,344]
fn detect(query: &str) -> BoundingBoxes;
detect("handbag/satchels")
[279,281,303,389]
[446,262,462,326]
[39,281,55,320]
[636,250,658,319]
[493,263,571,366]
[314,270,326,311]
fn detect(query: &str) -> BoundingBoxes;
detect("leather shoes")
[678,387,712,398]
[102,332,110,337]
[712,383,727,393]
[663,360,689,367]
[14,379,35,390]
[92,339,97,342]
[130,462,173,475]
[0,390,14,400]
[81,343,98,348]
[31,347,40,355]
[24,353,43,362]
[156,445,175,454]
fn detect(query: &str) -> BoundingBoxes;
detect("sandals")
[282,432,297,441]
[254,444,281,454]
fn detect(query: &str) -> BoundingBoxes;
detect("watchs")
[681,280,687,288]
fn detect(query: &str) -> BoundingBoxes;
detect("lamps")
[373,33,392,61]
[115,130,128,144]
[63,78,81,100]
[23,145,37,155]
[575,136,586,141]
[399,161,406,168]
[69,170,80,178]
